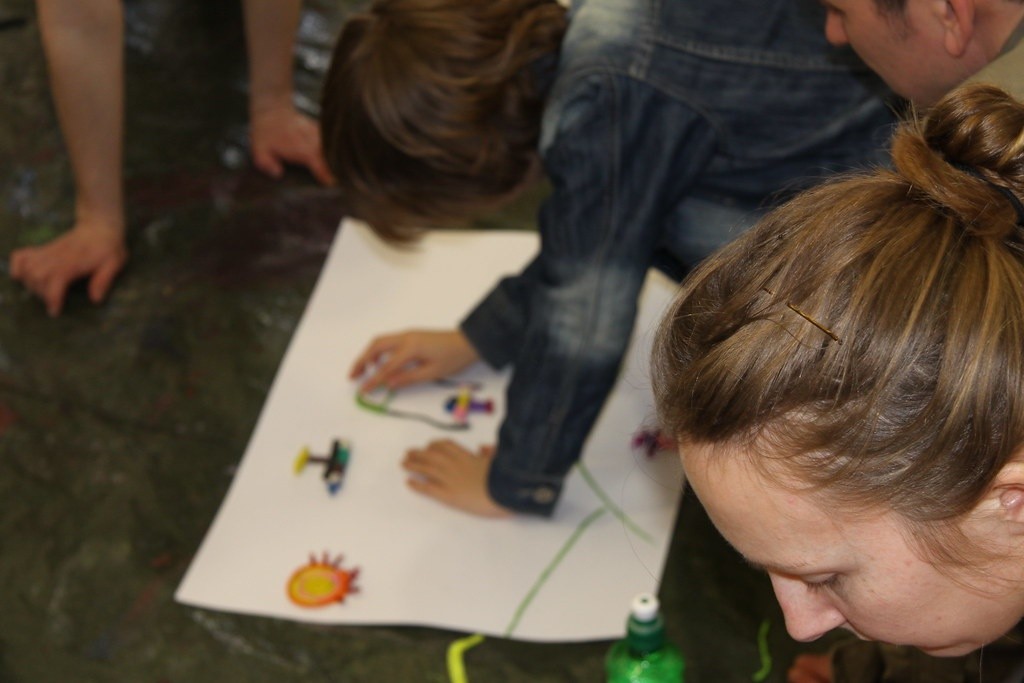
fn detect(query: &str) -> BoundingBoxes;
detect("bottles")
[605,593,689,683]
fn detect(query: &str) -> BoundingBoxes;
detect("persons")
[318,0,919,521]
[8,0,330,314]
[649,83,1023,683]
[826,0,1024,108]
